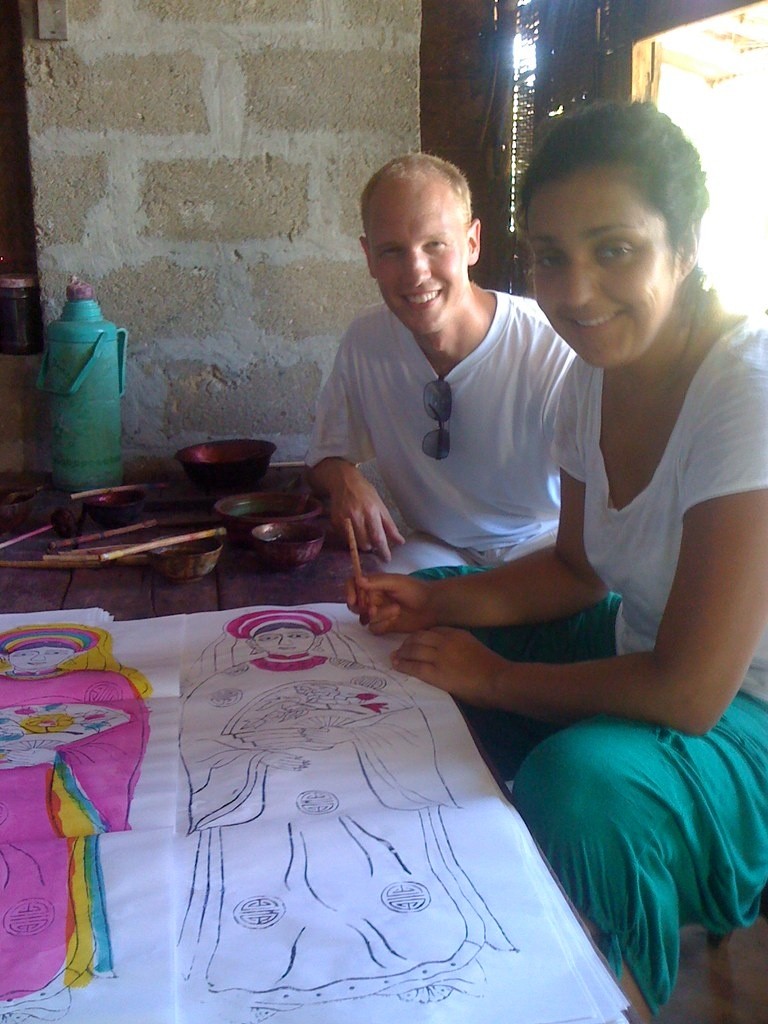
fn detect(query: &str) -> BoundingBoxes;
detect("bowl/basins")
[147,535,224,583]
[176,439,276,490]
[1,490,37,537]
[83,490,145,525]
[251,521,326,573]
[214,492,323,543]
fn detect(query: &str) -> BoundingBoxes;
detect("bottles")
[0,273,41,355]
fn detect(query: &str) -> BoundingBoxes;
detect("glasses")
[422,375,451,460]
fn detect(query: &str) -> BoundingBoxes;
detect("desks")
[0,467,391,623]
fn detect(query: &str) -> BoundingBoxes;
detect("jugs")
[41,282,131,491]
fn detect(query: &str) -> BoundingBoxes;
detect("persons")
[306,96,768,1024]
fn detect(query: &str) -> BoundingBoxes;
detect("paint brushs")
[268,460,306,468]
[292,491,311,514]
[0,525,54,548]
[98,527,228,563]
[70,481,172,499]
[342,516,370,626]
[0,518,158,569]
